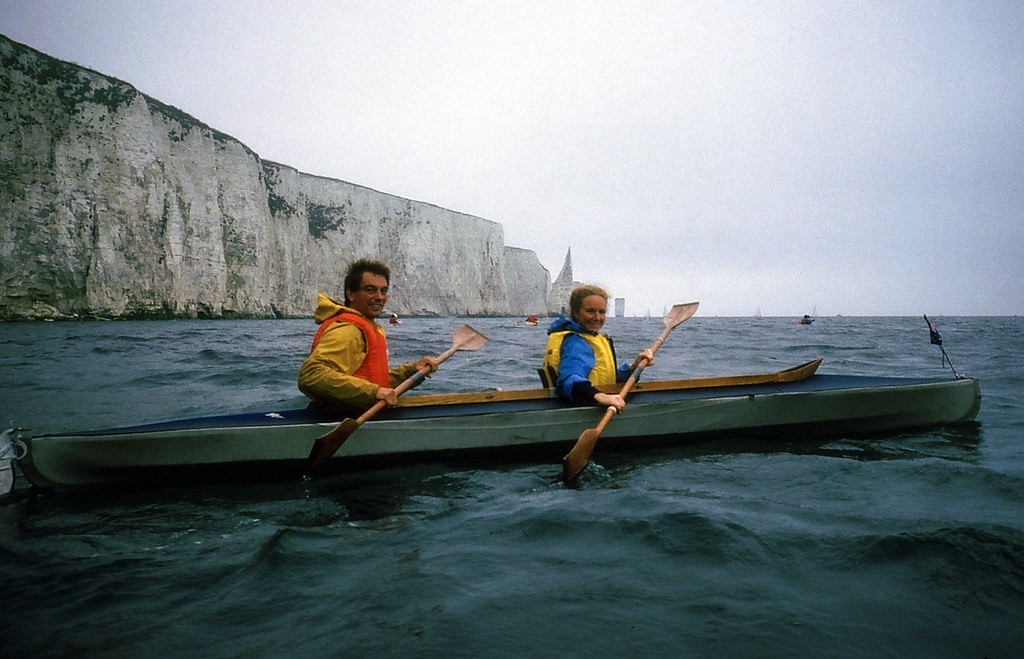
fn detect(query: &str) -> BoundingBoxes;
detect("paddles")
[516,317,544,323]
[306,324,490,471]
[563,302,700,486]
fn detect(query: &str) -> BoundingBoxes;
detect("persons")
[389,315,398,324]
[526,312,537,323]
[298,256,439,411]
[546,285,656,415]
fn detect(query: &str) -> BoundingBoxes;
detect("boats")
[525,321,539,326]
[0,358,982,508]
[801,318,815,324]
[388,319,402,326]
